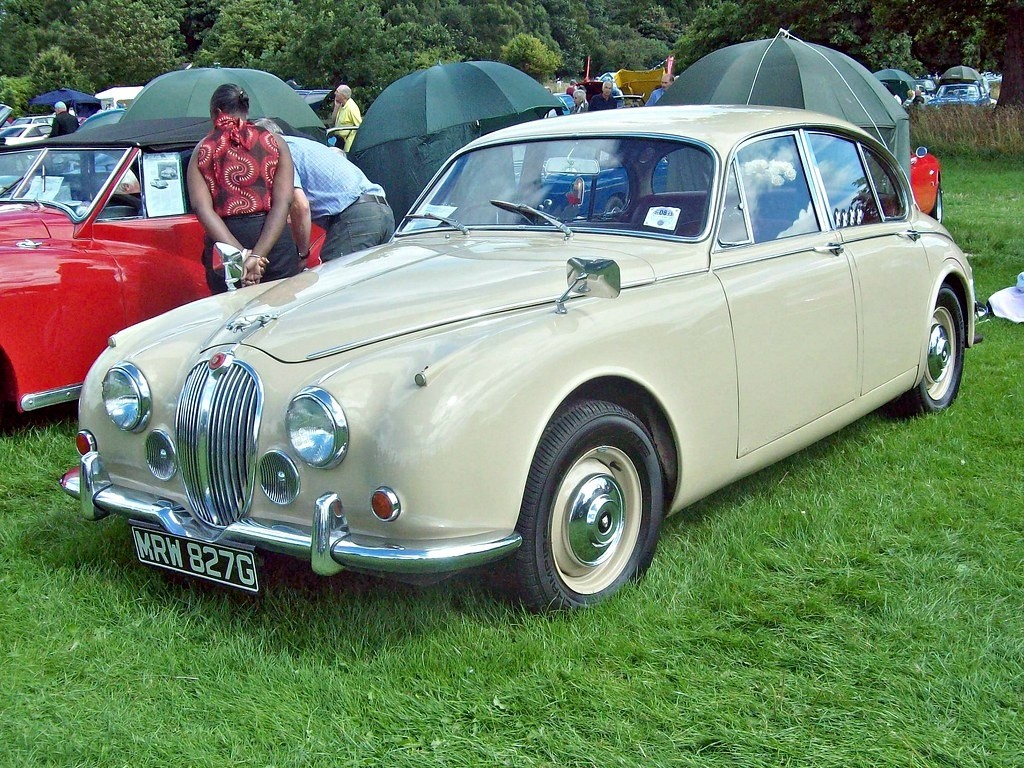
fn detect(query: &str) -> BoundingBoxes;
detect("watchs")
[298,250,310,260]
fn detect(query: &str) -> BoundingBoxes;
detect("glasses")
[573,97,580,99]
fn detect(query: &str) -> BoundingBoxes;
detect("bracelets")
[247,255,263,259]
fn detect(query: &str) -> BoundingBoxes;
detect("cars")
[57,94,984,623]
[0,113,348,434]
[1,67,1009,235]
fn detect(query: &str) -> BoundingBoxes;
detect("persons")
[560,77,618,116]
[47,101,80,138]
[248,116,397,270]
[185,84,301,295]
[643,76,676,108]
[330,85,362,156]
[903,89,925,109]
[105,102,123,111]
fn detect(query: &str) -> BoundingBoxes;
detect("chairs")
[629,189,708,238]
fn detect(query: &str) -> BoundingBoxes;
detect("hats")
[915,90,921,96]
[287,80,302,89]
[578,85,586,91]
[570,79,578,84]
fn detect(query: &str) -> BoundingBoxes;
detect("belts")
[352,194,386,204]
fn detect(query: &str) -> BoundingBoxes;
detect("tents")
[871,69,917,105]
[118,62,327,159]
[94,85,145,112]
[28,88,101,119]
[939,66,984,87]
[653,28,911,206]
[349,60,561,233]
